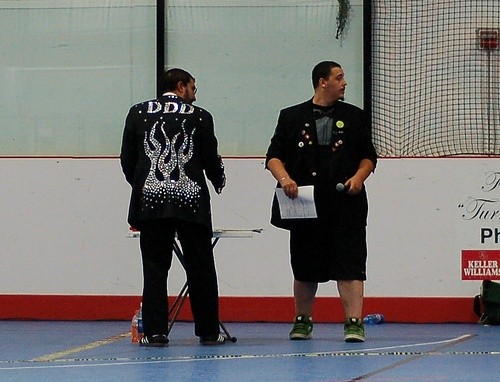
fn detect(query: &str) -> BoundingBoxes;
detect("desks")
[127,230,253,342]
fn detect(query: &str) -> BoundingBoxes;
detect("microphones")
[336,182,350,192]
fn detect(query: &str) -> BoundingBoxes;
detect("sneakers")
[139,334,169,347]
[200,333,225,345]
[289,314,314,338]
[343,318,365,341]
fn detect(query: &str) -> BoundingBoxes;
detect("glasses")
[182,82,197,93]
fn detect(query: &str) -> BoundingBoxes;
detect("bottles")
[130,310,139,343]
[138,301,144,343]
[363,314,384,325]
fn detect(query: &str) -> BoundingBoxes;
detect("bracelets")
[278,177,290,182]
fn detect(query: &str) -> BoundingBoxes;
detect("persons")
[266,61,377,343]
[120,69,227,347]
